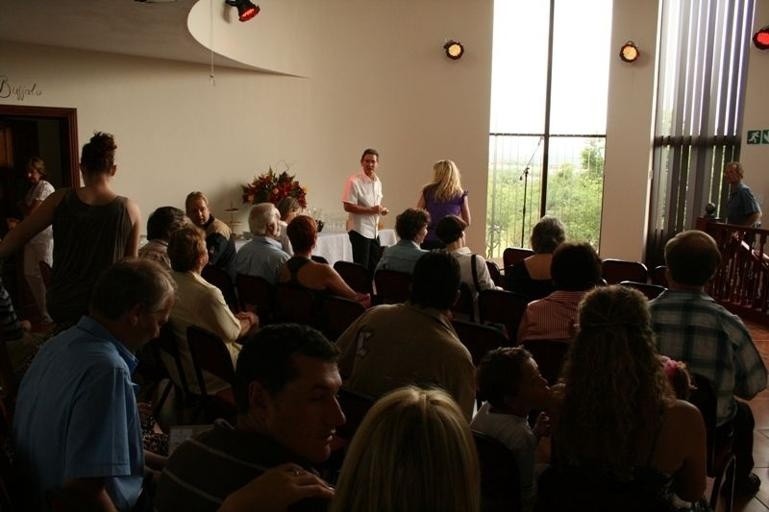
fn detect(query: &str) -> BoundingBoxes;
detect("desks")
[235,230,396,268]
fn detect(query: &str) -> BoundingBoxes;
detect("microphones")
[537,136,544,146]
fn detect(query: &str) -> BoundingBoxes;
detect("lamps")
[620,41,639,63]
[752,25,769,49]
[225,0,260,22]
[444,40,464,60]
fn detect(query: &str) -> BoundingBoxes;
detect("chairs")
[0,248,738,512]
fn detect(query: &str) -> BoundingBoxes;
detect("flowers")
[241,165,309,209]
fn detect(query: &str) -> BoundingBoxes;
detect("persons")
[342,149,388,282]
[415,159,471,247]
[7,154,57,332]
[8,189,766,510]
[2,134,138,339]
[721,160,762,248]
